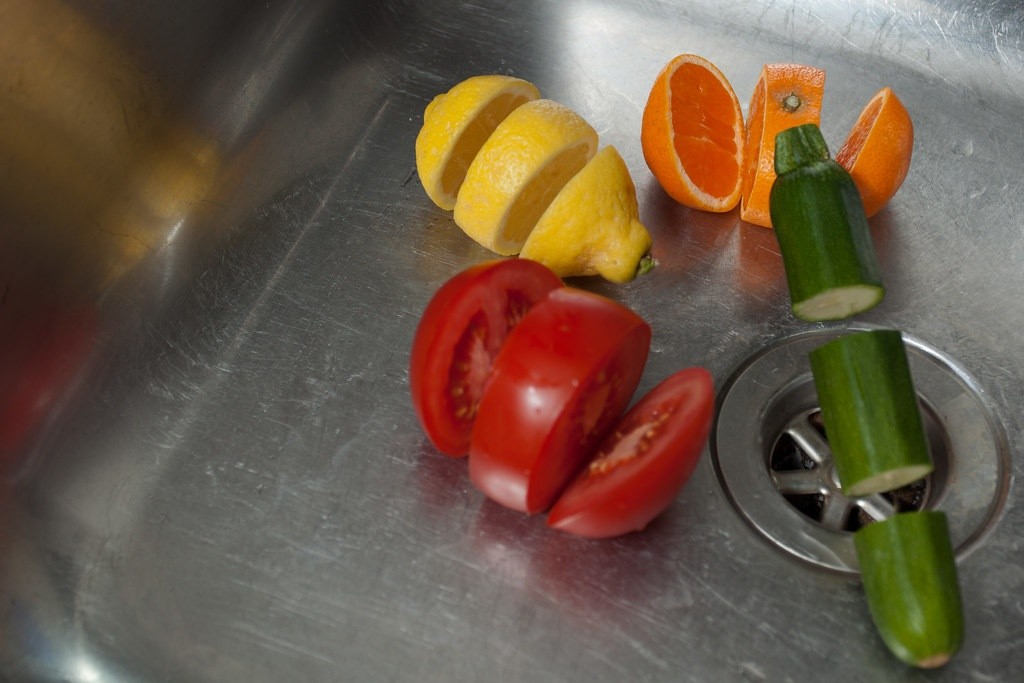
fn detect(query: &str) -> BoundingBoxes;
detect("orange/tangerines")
[642,53,913,227]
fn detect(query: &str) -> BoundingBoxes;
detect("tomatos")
[410,257,711,537]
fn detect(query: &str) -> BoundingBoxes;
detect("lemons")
[415,75,662,281]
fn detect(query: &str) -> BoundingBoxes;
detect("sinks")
[0,1,1024,683]
